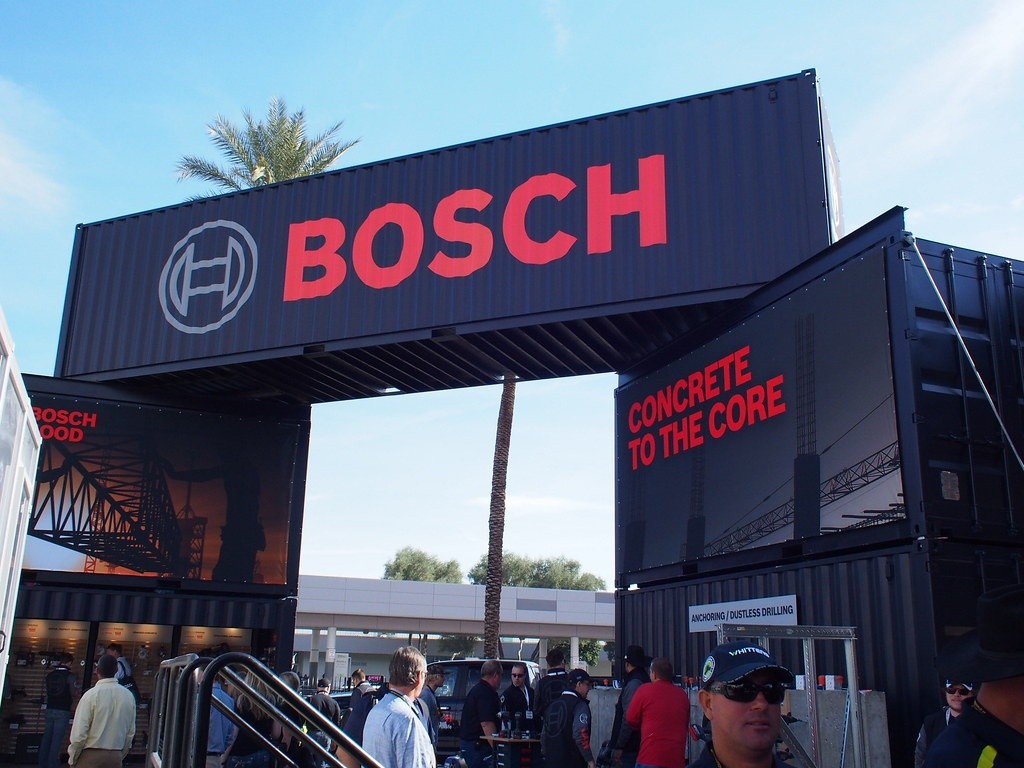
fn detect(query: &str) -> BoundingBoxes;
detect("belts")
[206,752,221,756]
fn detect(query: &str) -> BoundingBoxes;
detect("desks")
[480,736,540,768]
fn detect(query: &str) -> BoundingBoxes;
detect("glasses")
[942,686,970,695]
[511,674,526,678]
[701,678,785,704]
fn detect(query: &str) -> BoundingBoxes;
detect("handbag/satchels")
[118,661,142,705]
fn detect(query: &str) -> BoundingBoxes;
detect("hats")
[939,589,1024,684]
[94,654,118,676]
[701,641,796,689]
[566,668,593,687]
[624,645,654,669]
[317,678,331,687]
[427,666,452,675]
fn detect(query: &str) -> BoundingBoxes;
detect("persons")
[305,679,341,768]
[337,685,436,768]
[107,644,130,681]
[539,668,597,768]
[924,596,1024,768]
[37,653,80,768]
[532,649,569,717]
[348,669,371,708]
[420,666,450,743]
[915,680,980,768]
[626,657,690,768]
[194,649,234,768]
[460,661,502,768]
[688,640,794,768]
[223,670,315,768]
[501,664,535,730]
[68,655,137,768]
[361,646,436,768]
[609,646,653,768]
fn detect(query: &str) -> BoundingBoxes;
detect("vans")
[423,658,540,762]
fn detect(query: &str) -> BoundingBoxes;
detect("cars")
[329,690,379,724]
